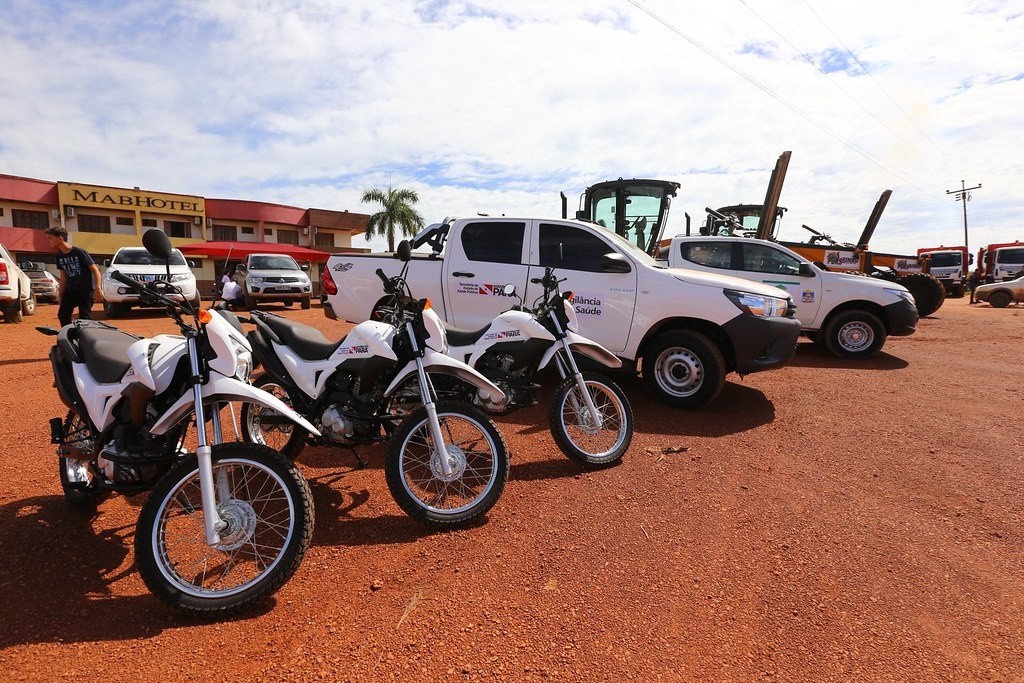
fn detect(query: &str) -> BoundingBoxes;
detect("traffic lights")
[970,253,973,264]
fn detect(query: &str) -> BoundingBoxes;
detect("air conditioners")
[67,207,76,218]
[314,227,317,234]
[207,218,212,227]
[52,210,61,221]
[195,217,200,225]
[304,228,309,234]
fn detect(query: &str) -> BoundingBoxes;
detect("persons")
[220,278,243,310]
[44,227,104,327]
[221,270,231,289]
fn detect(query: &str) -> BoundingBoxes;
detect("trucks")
[977,240,1024,284]
[917,245,968,299]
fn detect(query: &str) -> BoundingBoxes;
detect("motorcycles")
[237,240,510,529]
[382,242,634,467]
[36,228,322,616]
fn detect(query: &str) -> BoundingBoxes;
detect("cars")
[974,276,1024,308]
[102,247,200,318]
[21,269,60,305]
[231,254,312,310]
[0,243,36,323]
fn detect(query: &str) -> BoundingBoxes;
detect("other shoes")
[970,301,977,304]
[976,300,983,303]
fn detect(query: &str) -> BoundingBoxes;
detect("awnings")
[175,241,330,262]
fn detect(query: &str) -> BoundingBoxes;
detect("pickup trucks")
[320,216,802,409]
[655,236,919,359]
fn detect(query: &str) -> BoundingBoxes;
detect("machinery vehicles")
[560,151,946,317]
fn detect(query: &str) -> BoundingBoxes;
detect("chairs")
[551,241,563,265]
